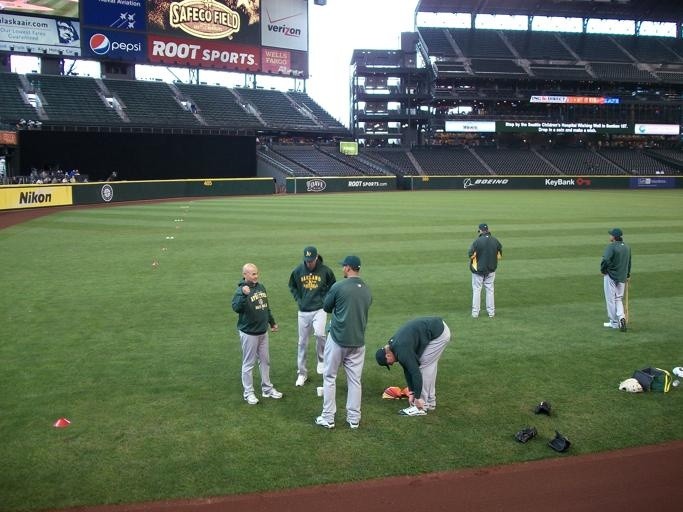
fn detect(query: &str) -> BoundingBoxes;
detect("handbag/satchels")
[631,367,672,393]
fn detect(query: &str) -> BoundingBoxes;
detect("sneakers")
[345,417,359,429]
[246,394,259,405]
[261,388,282,399]
[398,405,427,416]
[295,376,307,386]
[618,316,626,332]
[314,416,334,429]
[603,323,611,327]
[316,362,323,374]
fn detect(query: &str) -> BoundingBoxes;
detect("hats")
[303,246,316,262]
[607,228,622,237]
[375,348,389,370]
[338,256,360,268]
[477,224,487,232]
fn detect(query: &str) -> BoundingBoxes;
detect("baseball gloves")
[514,427,537,442]
[534,400,551,414]
[546,430,570,453]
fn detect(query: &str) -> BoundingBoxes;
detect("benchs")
[258,142,682,176]
[1,71,347,135]
[415,26,682,80]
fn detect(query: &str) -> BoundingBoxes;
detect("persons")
[314,255,372,429]
[375,315,451,417]
[468,222,503,319]
[600,228,631,332]
[31,167,80,184]
[191,103,198,114]
[231,263,284,405]
[288,246,336,388]
